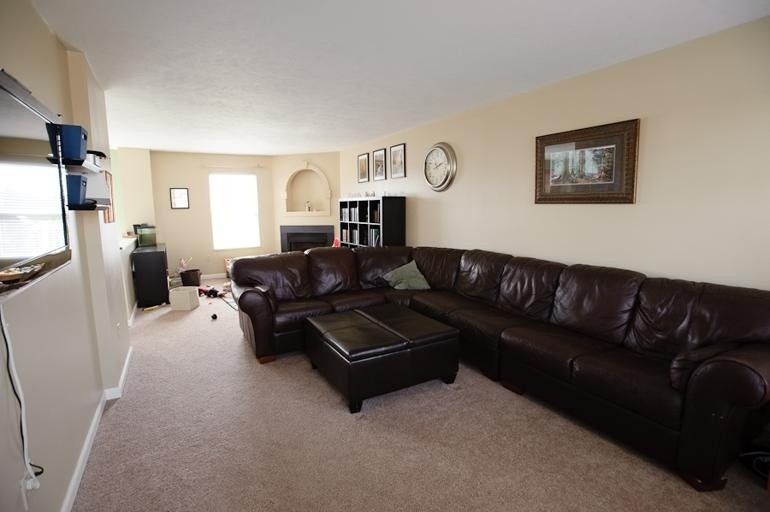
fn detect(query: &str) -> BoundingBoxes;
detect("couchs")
[229,246,769,492]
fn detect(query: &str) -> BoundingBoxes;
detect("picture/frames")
[535,118,636,204]
[390,143,406,178]
[170,188,189,209]
[100,171,115,223]
[357,153,370,183]
[373,148,387,180]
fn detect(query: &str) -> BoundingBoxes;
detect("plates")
[0,262,45,282]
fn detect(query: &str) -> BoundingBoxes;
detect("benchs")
[305,302,462,414]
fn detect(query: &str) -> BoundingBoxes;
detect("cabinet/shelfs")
[60,158,110,211]
[131,243,170,311]
[338,196,406,248]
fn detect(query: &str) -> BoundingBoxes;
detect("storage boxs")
[169,286,200,311]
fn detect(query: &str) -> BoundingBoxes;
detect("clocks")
[423,143,457,192]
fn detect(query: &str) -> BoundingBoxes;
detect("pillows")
[382,260,430,290]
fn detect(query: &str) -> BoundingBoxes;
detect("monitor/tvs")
[0,68,73,308]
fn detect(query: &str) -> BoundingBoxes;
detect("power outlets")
[20,469,38,494]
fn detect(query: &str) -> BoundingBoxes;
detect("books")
[370,203,380,248]
[341,207,359,244]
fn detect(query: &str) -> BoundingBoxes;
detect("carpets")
[202,278,238,310]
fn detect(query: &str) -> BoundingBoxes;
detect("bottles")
[305,201,311,211]
